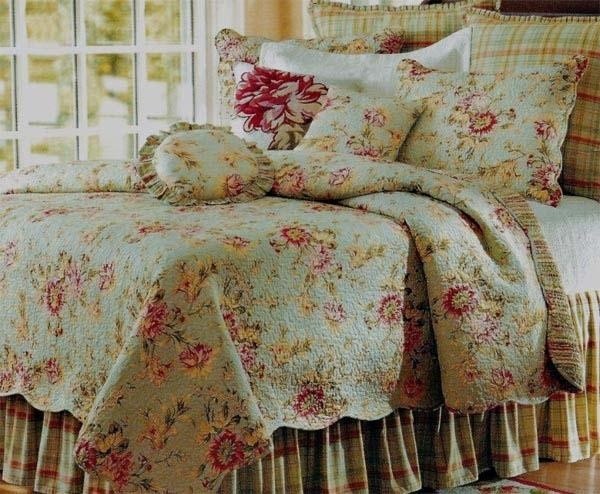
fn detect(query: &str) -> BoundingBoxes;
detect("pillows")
[231,58,368,159]
[394,56,588,206]
[307,1,500,49]
[134,123,277,206]
[465,8,599,192]
[261,26,472,95]
[214,28,405,128]
[295,81,416,164]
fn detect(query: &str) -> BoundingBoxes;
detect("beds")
[1,160,600,494]
